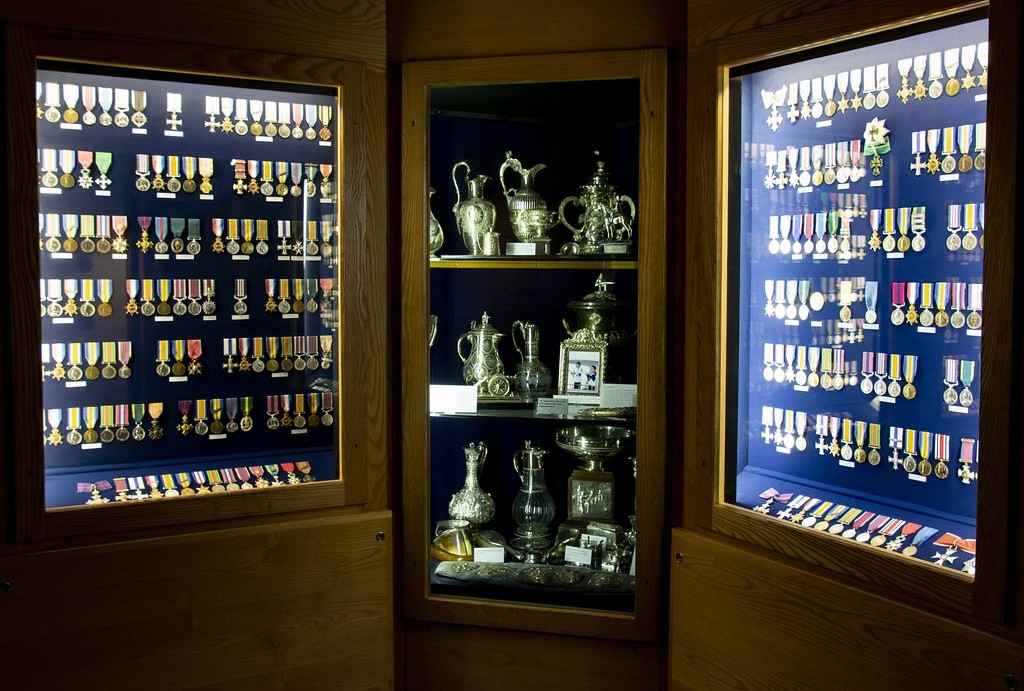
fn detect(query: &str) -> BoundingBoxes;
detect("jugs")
[510,318,550,398]
[448,439,498,533]
[450,161,498,254]
[513,440,557,540]
[558,162,637,243]
[498,149,560,240]
[457,310,507,392]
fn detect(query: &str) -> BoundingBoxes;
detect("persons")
[585,365,596,390]
[569,360,583,390]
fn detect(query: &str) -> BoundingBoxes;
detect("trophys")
[553,426,636,526]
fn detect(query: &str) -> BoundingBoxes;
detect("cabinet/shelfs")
[683,0,1024,628]
[6,39,370,544]
[401,44,673,641]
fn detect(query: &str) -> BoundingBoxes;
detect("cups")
[509,207,560,244]
